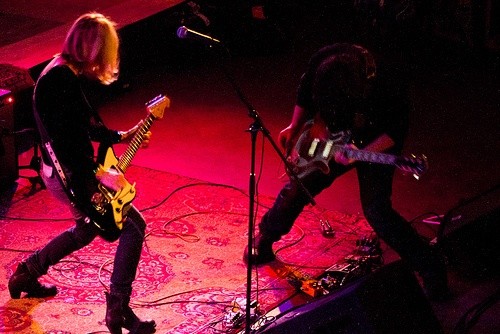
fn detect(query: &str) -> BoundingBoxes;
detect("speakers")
[443,184,500,283]
[260,258,443,334]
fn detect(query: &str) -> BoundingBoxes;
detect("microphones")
[177,26,224,47]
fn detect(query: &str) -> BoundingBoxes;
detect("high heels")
[103,291,157,334]
[8,262,57,299]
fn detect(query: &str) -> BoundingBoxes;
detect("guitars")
[90,94,170,243]
[286,119,429,184]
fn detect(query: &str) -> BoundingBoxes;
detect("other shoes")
[242,233,275,264]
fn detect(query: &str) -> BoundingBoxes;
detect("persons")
[7,12,157,334]
[243,42,453,297]
